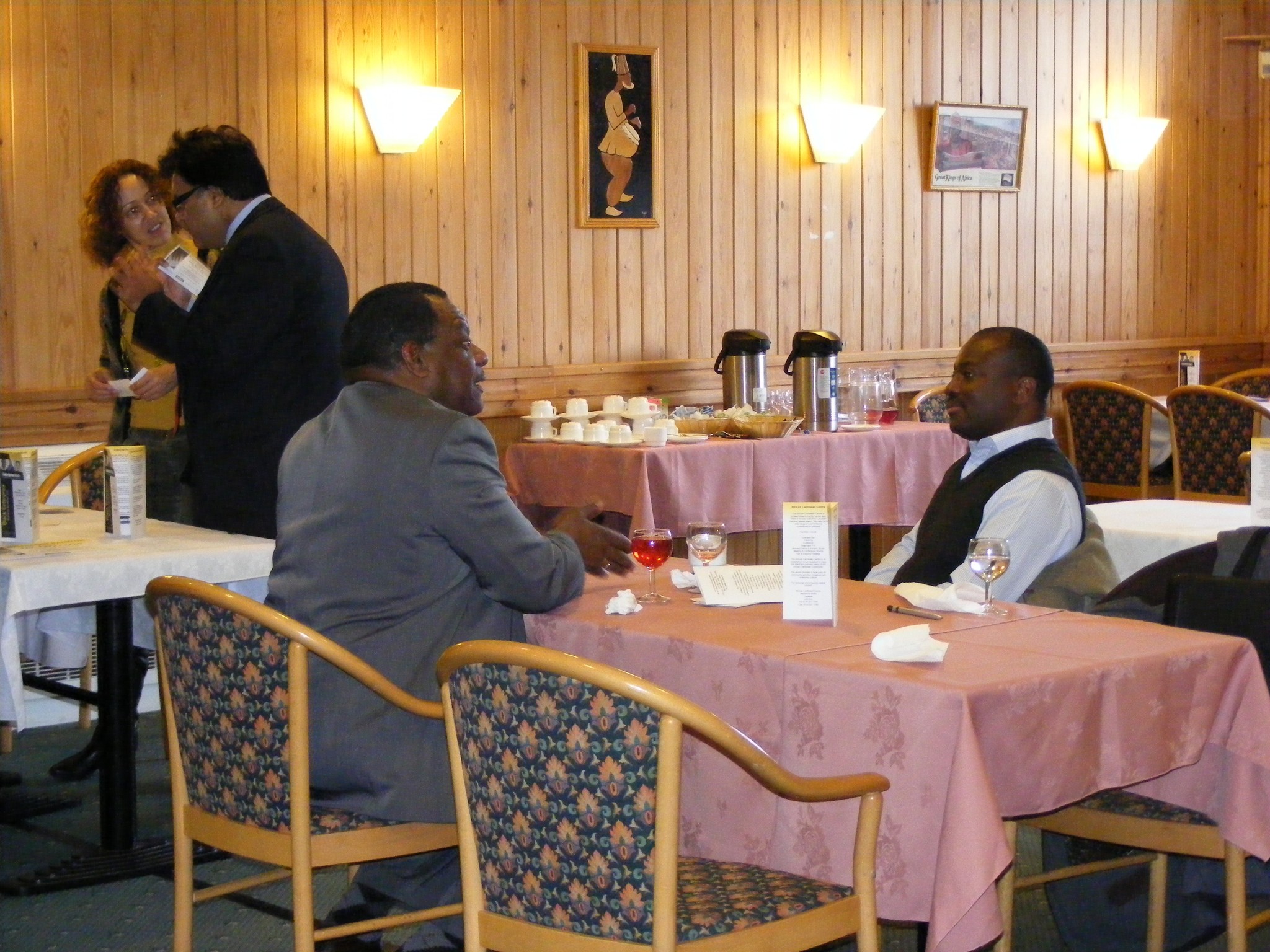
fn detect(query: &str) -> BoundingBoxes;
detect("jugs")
[783,330,843,434]
[714,330,772,413]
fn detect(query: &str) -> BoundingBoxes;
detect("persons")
[860,325,1086,617]
[51,158,222,783]
[107,124,350,544]
[257,280,633,951]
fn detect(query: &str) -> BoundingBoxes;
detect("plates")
[520,410,710,445]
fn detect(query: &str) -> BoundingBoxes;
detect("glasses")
[170,184,204,212]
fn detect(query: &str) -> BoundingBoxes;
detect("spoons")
[667,433,713,437]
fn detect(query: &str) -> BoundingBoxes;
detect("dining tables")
[492,417,983,587]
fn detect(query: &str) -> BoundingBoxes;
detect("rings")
[605,563,611,569]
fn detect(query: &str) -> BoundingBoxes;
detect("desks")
[1134,394,1270,478]
[489,549,1270,952]
[0,490,279,858]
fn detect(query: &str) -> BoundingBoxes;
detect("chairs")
[906,384,956,429]
[136,571,462,952]
[1205,368,1269,401]
[28,444,140,727]
[419,639,898,951]
[1159,382,1269,510]
[997,521,1270,952]
[1059,378,1179,505]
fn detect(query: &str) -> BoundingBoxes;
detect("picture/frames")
[924,100,1030,194]
[574,39,666,230]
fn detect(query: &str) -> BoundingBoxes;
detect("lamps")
[796,97,888,166]
[1098,116,1172,171]
[352,79,462,155]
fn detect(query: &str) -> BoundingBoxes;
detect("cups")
[836,365,898,424]
[530,395,677,446]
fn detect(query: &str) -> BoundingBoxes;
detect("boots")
[47,646,150,782]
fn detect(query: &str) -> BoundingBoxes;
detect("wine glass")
[632,529,674,604]
[685,522,728,593]
[968,538,1011,615]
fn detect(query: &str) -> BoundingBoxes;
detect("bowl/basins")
[736,414,806,438]
[674,417,732,433]
